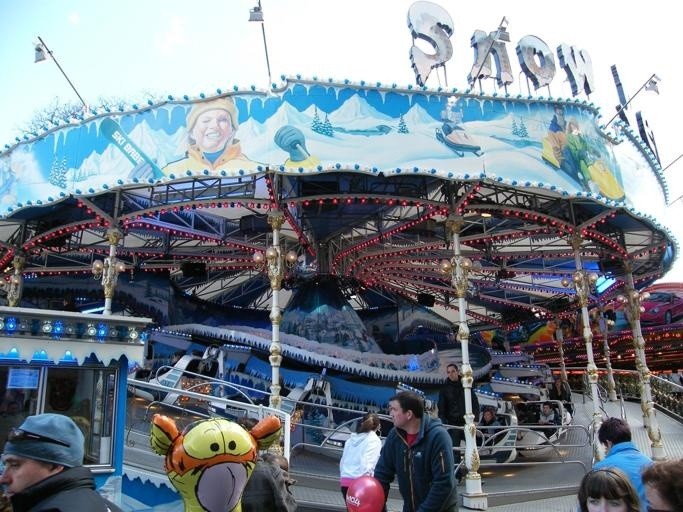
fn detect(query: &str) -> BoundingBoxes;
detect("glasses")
[6,426,70,448]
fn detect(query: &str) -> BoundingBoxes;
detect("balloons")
[148,409,285,512]
[344,475,384,512]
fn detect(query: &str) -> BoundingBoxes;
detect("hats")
[4,412,86,468]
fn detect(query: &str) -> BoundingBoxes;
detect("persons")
[561,115,601,170]
[577,465,642,512]
[547,377,572,417]
[438,103,466,136]
[58,292,81,314]
[530,401,563,441]
[641,459,682,511]
[588,416,659,512]
[435,362,485,485]
[134,351,182,379]
[123,92,320,180]
[475,407,504,447]
[370,390,459,511]
[513,401,538,423]
[546,101,580,184]
[337,409,390,512]
[666,365,683,419]
[0,412,125,512]
